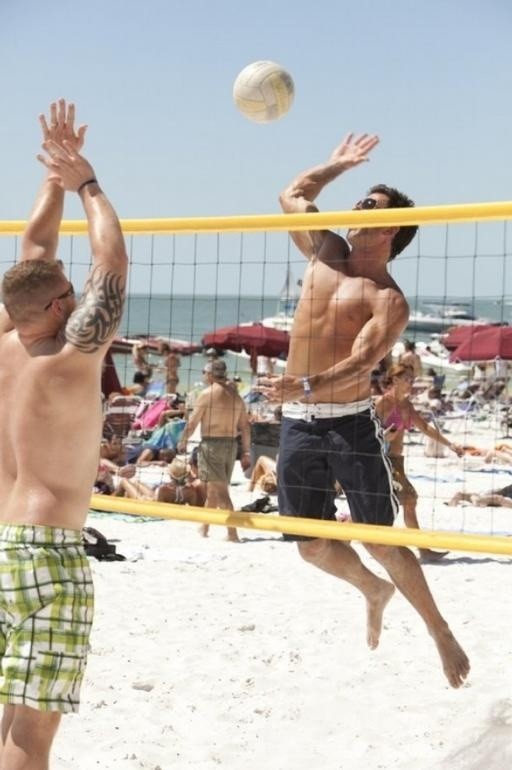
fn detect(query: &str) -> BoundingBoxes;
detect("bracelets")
[73,177,99,199]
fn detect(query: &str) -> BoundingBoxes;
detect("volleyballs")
[234,62,293,121]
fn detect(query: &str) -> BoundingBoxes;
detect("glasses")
[357,198,376,209]
[44,281,74,311]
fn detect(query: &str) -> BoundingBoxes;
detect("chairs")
[104,381,177,443]
[376,360,511,460]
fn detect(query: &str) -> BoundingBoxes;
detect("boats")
[406,298,492,335]
[225,283,302,368]
[110,334,203,358]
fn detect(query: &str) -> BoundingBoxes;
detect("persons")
[250,127,474,693]
[3,95,132,770]
[86,313,511,566]
